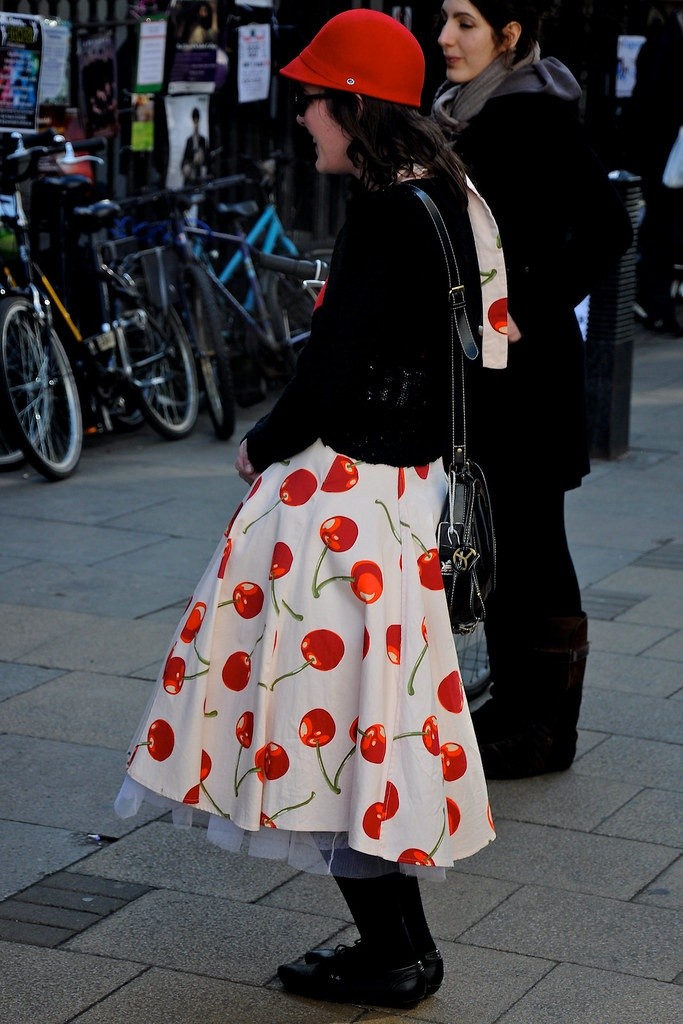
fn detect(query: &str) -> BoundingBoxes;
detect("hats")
[279,10,426,107]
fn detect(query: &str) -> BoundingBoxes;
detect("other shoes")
[470,701,577,779]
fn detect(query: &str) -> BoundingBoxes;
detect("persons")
[182,109,206,187]
[430,0,591,780]
[132,94,150,121]
[630,10,683,337]
[176,0,213,43]
[112,9,508,1009]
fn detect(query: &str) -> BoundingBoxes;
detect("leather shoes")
[277,945,425,1011]
[305,937,444,999]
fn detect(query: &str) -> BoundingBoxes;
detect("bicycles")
[0,122,332,483]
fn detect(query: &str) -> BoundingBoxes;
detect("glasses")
[295,92,327,116]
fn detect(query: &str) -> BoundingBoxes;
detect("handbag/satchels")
[436,460,497,634]
